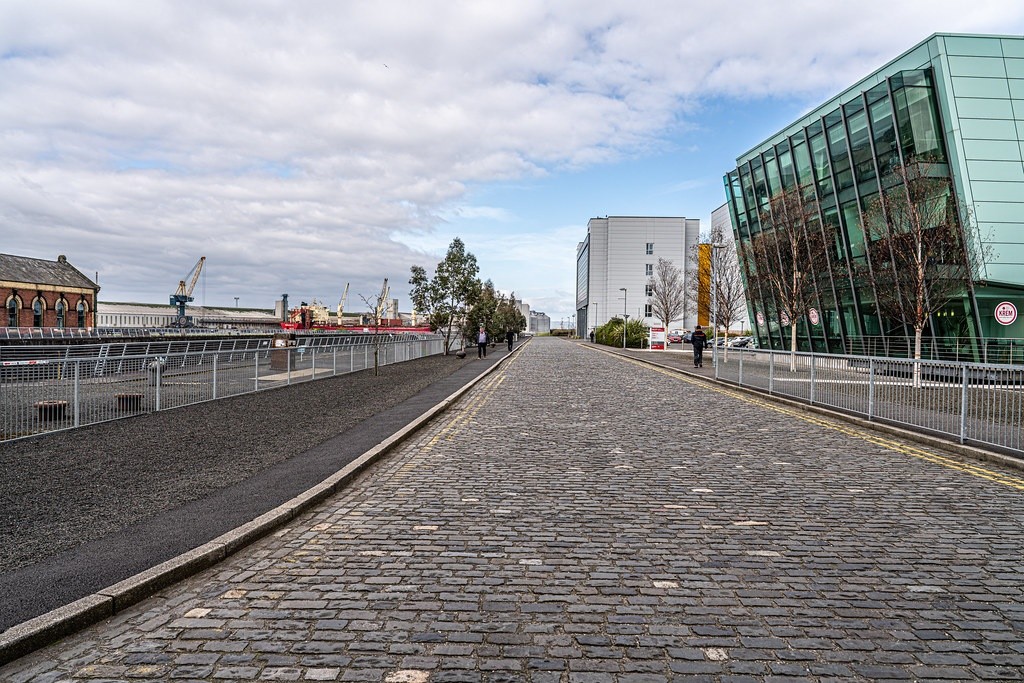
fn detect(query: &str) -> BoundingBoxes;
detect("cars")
[681,333,759,348]
[667,334,681,344]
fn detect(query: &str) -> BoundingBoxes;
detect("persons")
[475,326,490,360]
[506,328,515,351]
[691,325,707,368]
[590,331,594,343]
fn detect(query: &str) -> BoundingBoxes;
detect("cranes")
[377,277,391,325]
[337,282,350,325]
[168,256,206,320]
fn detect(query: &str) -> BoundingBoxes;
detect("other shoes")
[478,357,480,360]
[699,363,702,367]
[694,366,698,368]
[484,356,487,359]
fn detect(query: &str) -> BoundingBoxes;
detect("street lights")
[619,288,627,347]
[234,297,240,308]
[741,320,745,332]
[593,302,598,329]
[713,242,728,381]
